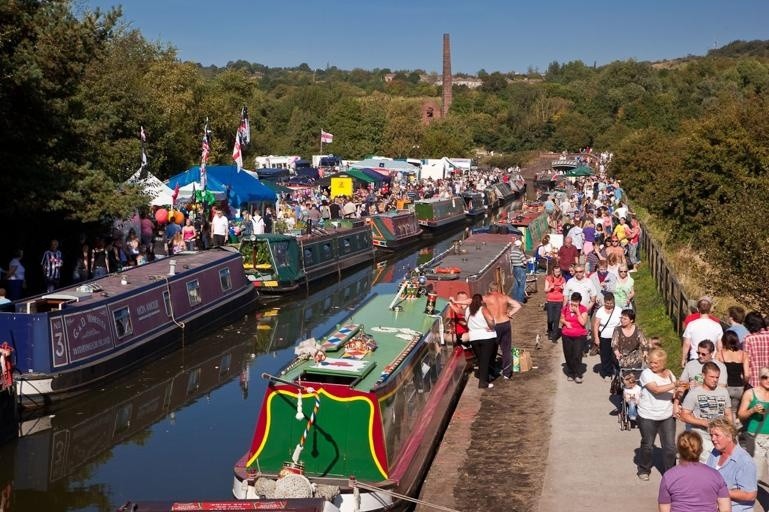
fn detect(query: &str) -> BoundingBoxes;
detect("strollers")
[617,356,648,432]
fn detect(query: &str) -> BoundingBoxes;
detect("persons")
[604,236,626,264]
[545,147,636,231]
[242,166,521,236]
[743,312,769,390]
[636,349,686,480]
[210,210,229,247]
[657,430,732,512]
[681,300,724,368]
[607,253,619,275]
[738,367,769,483]
[683,295,721,333]
[715,330,750,410]
[706,416,757,512]
[566,220,585,263]
[589,260,616,356]
[582,220,595,259]
[626,217,640,272]
[611,309,647,368]
[544,266,566,343]
[585,240,607,275]
[672,339,728,430]
[623,373,641,420]
[562,264,597,331]
[535,234,552,268]
[564,263,576,282]
[557,237,578,277]
[510,240,528,303]
[680,362,733,464]
[7,249,25,300]
[615,265,634,309]
[40,240,63,291]
[464,293,497,389]
[636,228,642,264]
[449,281,522,379]
[71,212,197,282]
[724,305,750,343]
[559,292,588,383]
[446,291,469,345]
[613,217,629,257]
[593,293,622,367]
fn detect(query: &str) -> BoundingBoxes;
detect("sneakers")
[504,375,509,379]
[487,383,494,389]
[575,377,582,383]
[638,473,649,480]
[567,376,573,381]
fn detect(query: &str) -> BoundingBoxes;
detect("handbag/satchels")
[519,349,533,372]
[739,412,765,457]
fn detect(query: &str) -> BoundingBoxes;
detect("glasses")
[619,316,628,320]
[619,270,627,273]
[612,240,618,242]
[696,350,709,356]
[761,375,769,380]
[575,271,583,273]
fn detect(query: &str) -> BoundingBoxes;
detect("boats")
[492,182,516,206]
[258,267,373,352]
[494,207,550,252]
[413,222,528,302]
[14,307,256,491]
[238,218,376,293]
[510,175,526,195]
[0,247,261,409]
[414,197,466,229]
[366,207,423,249]
[232,263,468,512]
[460,191,487,217]
[0,339,19,413]
[478,188,500,210]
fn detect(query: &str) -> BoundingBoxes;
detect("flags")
[320,130,333,144]
[200,124,210,189]
[232,106,251,174]
